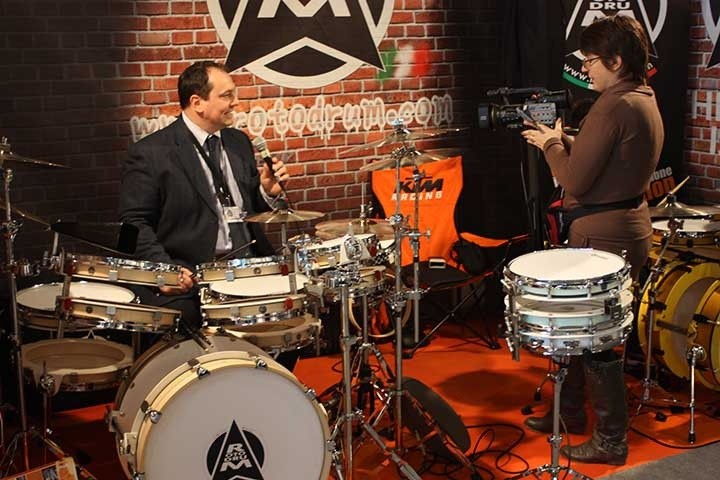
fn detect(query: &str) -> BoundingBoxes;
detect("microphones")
[482,81,539,99]
[252,137,286,195]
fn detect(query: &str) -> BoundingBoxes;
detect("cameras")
[475,88,577,138]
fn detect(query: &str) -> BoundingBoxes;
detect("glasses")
[583,55,611,67]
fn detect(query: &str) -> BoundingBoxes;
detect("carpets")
[0,312,720,480]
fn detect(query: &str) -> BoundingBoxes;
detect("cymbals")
[359,147,465,174]
[243,208,326,223]
[0,197,51,227]
[313,217,396,244]
[340,126,473,156]
[0,149,73,170]
[650,217,720,235]
[649,202,720,218]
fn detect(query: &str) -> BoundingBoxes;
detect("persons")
[118,60,300,378]
[521,15,665,468]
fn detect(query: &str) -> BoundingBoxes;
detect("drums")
[636,255,720,393]
[55,251,186,290]
[14,338,135,394]
[53,294,181,336]
[195,232,412,352]
[14,279,141,333]
[501,248,635,358]
[105,325,337,480]
[649,229,720,270]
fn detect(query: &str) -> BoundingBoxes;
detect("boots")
[557,358,628,466]
[523,367,587,434]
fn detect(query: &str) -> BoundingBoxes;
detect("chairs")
[367,155,533,359]
